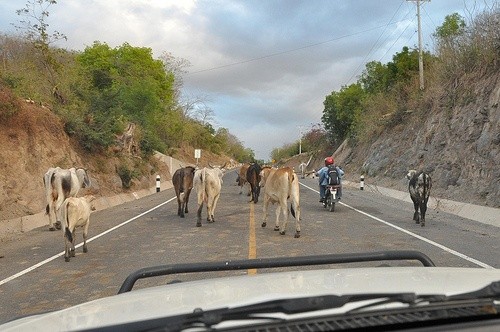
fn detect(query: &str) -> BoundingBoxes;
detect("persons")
[315,157,344,202]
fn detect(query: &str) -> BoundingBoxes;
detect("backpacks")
[326,165,340,185]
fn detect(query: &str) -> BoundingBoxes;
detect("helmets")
[325,157,334,165]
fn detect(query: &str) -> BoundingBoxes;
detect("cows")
[407,169,432,227]
[299,162,307,180]
[234,162,303,239]
[43,166,97,263]
[172,158,228,227]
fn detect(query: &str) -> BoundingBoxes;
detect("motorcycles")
[316,174,342,212]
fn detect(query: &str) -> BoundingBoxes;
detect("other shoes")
[320,197,326,202]
[338,197,341,200]
